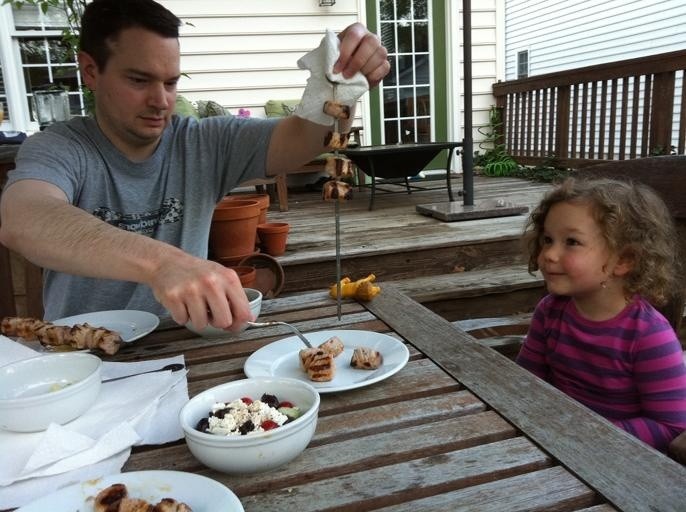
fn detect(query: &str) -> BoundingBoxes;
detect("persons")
[513,177,685,457]
[1,0,392,335]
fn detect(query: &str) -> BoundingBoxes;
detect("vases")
[210,192,290,298]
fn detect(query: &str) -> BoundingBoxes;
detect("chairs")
[172,95,365,211]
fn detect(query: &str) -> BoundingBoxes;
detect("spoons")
[103,362,187,384]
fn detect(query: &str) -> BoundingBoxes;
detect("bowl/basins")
[1,350,108,434]
[177,377,324,480]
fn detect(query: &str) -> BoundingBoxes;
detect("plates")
[13,307,162,353]
[10,468,243,512]
[239,327,410,395]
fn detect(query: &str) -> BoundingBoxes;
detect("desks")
[339,142,463,209]
